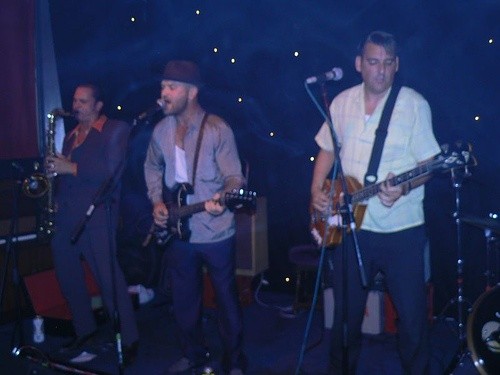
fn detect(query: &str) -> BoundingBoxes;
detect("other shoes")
[48,330,105,361]
[168,356,196,374]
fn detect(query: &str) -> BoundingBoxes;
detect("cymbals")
[461,216,500,234]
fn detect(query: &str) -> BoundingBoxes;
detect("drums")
[466,282,500,375]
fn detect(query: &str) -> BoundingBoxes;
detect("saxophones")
[22,109,76,240]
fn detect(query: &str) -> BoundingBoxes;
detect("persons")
[311,32,443,375]
[49,81,140,368]
[145,62,249,374]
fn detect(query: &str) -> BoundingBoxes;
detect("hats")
[152,61,207,89]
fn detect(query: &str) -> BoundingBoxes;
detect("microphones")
[307,67,344,85]
[135,98,166,123]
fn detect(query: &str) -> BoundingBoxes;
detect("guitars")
[151,182,257,248]
[308,138,472,249]
